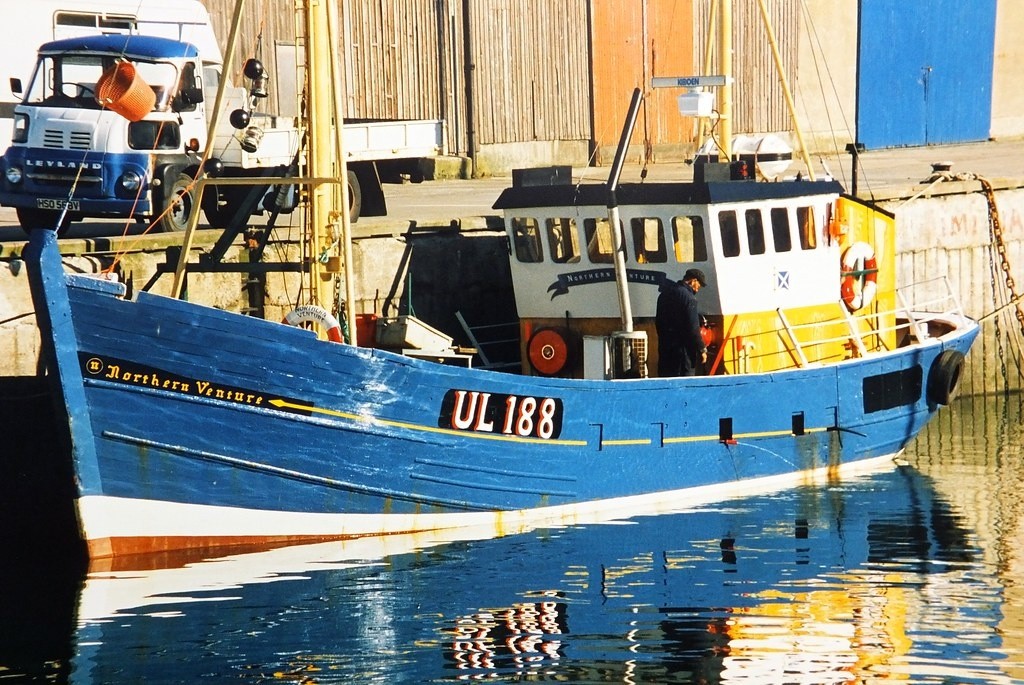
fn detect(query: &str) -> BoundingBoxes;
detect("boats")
[22,87,980,634]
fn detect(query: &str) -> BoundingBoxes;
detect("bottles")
[741,161,748,177]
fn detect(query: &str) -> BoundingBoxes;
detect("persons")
[655,269,708,378]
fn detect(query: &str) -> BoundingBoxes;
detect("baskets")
[95,58,156,122]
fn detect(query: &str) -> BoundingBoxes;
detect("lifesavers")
[841,241,877,310]
[280,304,344,343]
[927,349,966,405]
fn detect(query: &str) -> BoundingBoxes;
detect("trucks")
[0,34,448,239]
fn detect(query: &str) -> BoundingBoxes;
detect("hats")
[686,269,707,287]
